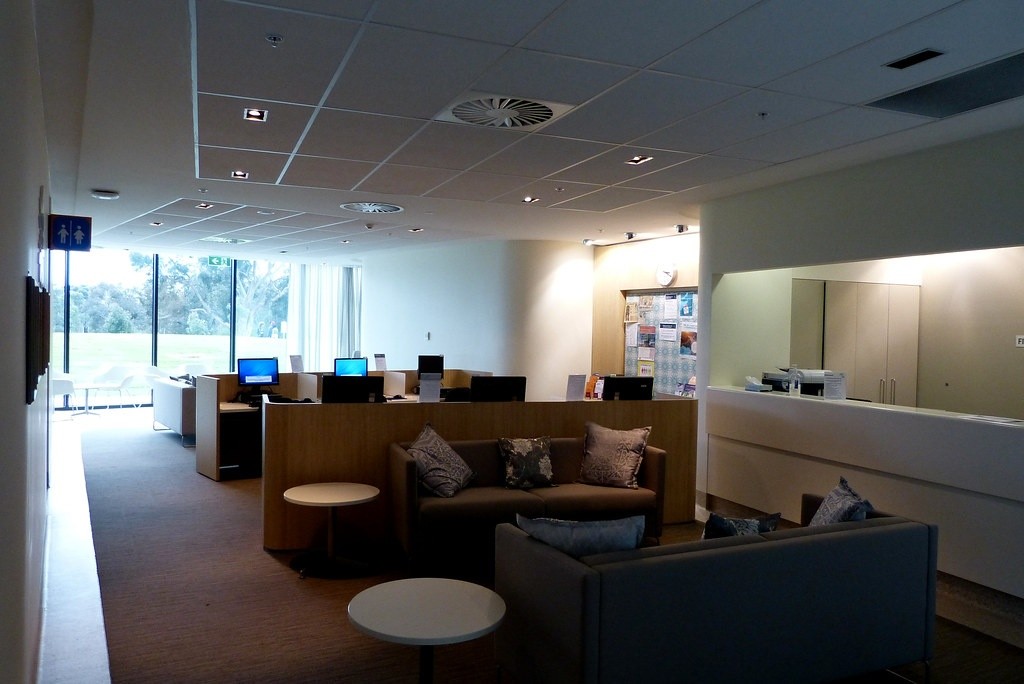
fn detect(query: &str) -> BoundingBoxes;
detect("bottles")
[788,365,800,397]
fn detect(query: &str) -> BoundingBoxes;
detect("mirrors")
[169,373,196,387]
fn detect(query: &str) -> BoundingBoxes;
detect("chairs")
[52,361,172,421]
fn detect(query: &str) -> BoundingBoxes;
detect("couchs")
[389,437,668,575]
[495,494,939,683]
[152,377,197,449]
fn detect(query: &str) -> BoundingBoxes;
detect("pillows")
[514,512,646,559]
[808,475,876,528]
[495,433,560,491]
[701,510,782,540]
[407,420,476,499]
[573,419,653,489]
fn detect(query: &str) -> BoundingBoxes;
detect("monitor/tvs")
[238,358,280,395]
[322,358,384,404]
[602,376,654,400]
[470,376,526,401]
[418,356,444,380]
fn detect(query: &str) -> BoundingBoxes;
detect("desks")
[196,370,700,552]
[72,384,101,416]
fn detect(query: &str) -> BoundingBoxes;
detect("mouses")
[303,398,311,403]
[394,395,402,398]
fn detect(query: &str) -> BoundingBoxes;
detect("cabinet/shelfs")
[789,280,857,399]
[854,282,919,407]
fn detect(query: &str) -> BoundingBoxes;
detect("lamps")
[675,224,689,233]
[655,263,679,286]
[624,232,637,240]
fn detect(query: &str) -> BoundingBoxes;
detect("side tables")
[348,578,506,684]
[282,482,381,579]
